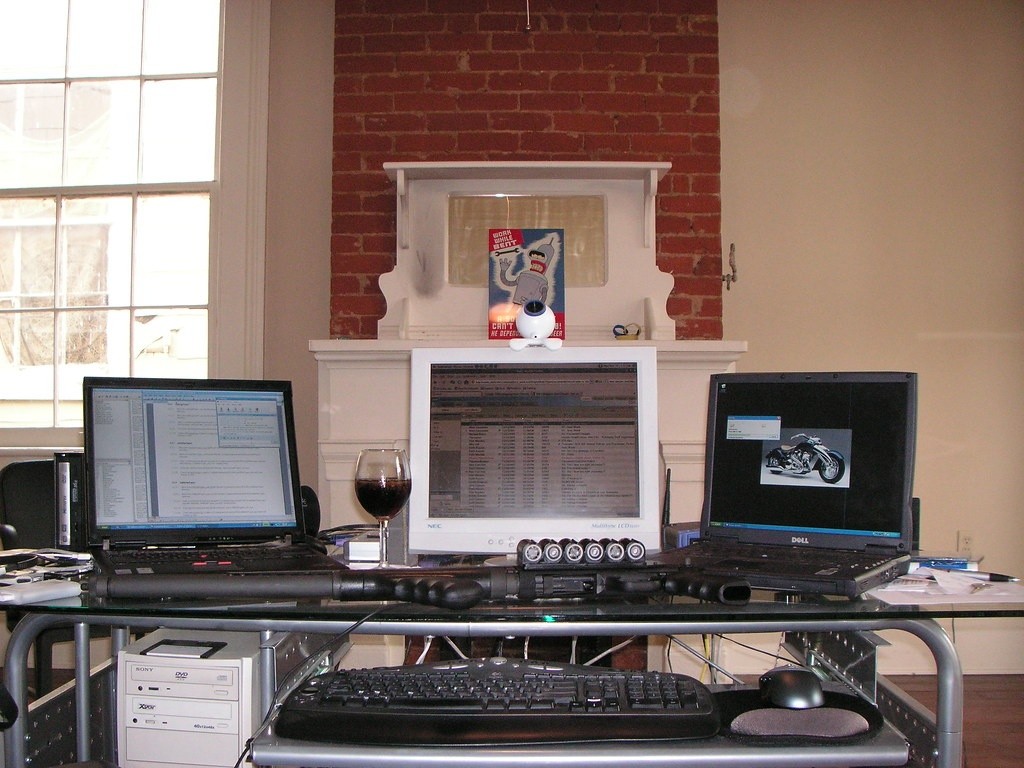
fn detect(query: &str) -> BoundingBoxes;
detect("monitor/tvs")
[410,346,661,565]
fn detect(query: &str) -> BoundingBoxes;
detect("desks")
[0,557,1024,768]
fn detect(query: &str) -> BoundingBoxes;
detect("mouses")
[758,664,825,709]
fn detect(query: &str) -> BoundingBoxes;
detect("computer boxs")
[117,626,261,768]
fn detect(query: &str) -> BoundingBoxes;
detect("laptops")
[83,378,350,575]
[648,371,917,596]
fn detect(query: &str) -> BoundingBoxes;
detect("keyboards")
[274,656,719,746]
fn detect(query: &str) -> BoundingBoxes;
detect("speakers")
[290,485,321,544]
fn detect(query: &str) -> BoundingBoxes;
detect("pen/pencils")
[932,569,1024,584]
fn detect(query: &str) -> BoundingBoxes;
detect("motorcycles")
[766,433,845,483]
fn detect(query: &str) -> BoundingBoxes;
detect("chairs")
[0,458,159,702]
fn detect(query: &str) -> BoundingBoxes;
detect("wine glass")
[354,448,413,569]
